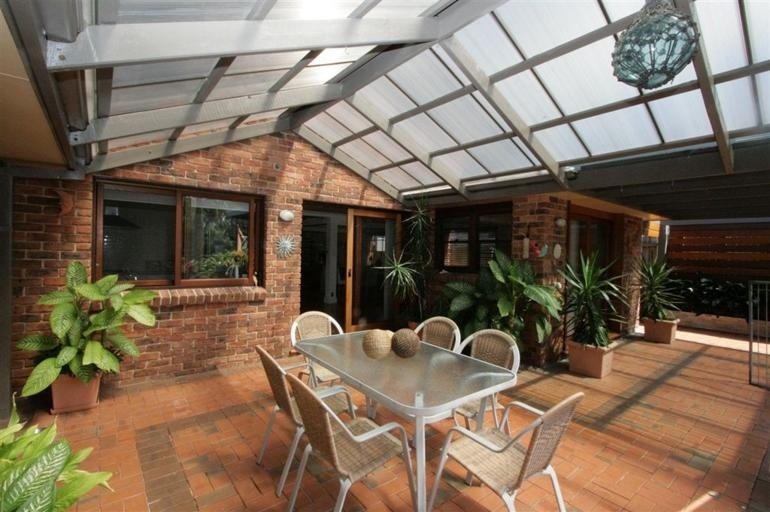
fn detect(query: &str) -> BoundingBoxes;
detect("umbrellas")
[104,215,141,228]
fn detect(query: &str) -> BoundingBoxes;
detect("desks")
[292,330,519,510]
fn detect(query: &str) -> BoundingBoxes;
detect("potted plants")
[12,258,160,415]
[555,249,624,377]
[381,188,437,337]
[636,252,680,345]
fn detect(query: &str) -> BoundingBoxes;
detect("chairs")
[255,344,360,496]
[425,391,585,512]
[398,317,462,417]
[283,373,421,512]
[285,311,370,420]
[428,330,525,485]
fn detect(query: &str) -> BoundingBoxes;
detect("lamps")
[609,1,704,92]
